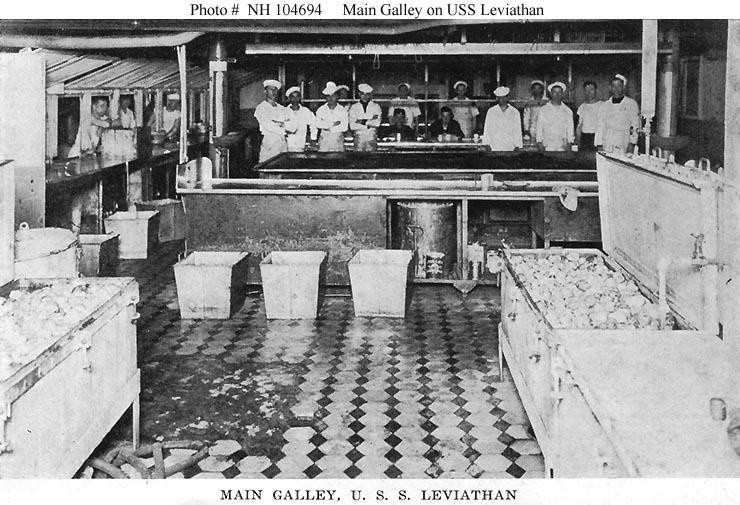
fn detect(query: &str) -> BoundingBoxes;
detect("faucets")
[682,230,717,271]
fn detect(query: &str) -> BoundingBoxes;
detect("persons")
[523,80,549,141]
[254,70,423,163]
[482,86,523,151]
[427,107,465,142]
[575,80,604,151]
[593,74,641,159]
[65,92,181,217]
[536,81,575,152]
[450,80,480,137]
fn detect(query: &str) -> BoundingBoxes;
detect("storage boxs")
[347,248,414,317]
[174,251,249,321]
[133,197,186,242]
[259,250,328,320]
[76,234,119,277]
[0,276,143,478]
[546,329,738,476]
[105,210,161,257]
[497,144,717,456]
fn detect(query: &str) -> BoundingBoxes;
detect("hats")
[322,82,349,96]
[454,80,468,89]
[532,80,566,93]
[262,79,301,98]
[358,84,374,94]
[397,82,411,91]
[615,74,627,86]
[167,94,179,101]
[493,86,510,97]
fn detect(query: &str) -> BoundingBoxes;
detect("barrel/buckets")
[396,201,456,278]
[14,221,82,278]
